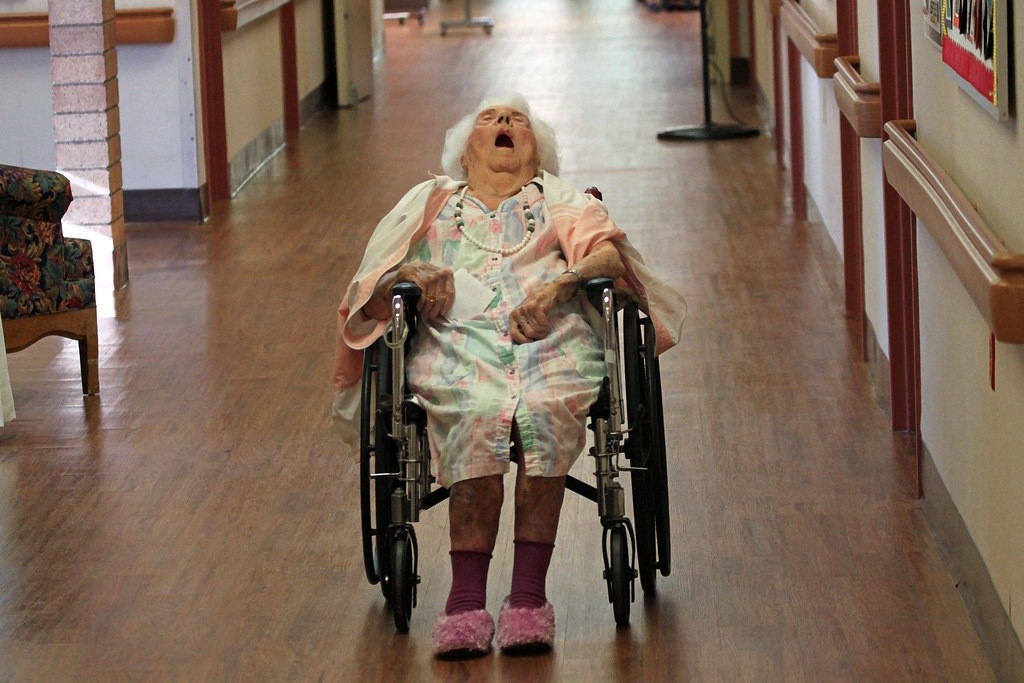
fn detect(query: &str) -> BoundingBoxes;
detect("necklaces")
[454,185,535,256]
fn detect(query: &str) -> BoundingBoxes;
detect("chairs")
[0,164,100,396]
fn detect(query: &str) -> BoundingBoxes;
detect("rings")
[426,295,435,305]
[517,320,528,333]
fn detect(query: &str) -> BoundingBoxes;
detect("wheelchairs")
[358,185,673,625]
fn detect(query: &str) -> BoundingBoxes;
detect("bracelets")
[562,268,582,303]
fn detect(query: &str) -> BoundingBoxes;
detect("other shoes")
[495,593,556,656]
[430,611,496,658]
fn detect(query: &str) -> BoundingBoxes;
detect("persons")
[334,92,687,657]
